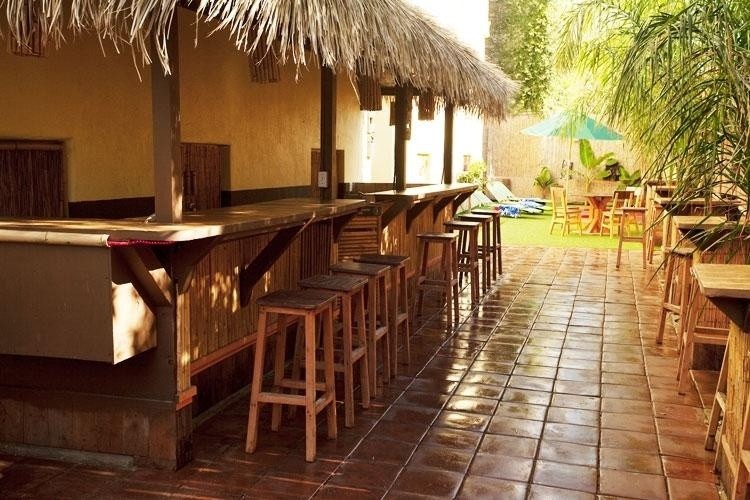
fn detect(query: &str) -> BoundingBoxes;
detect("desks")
[572,194,612,234]
[692,262,750,496]
[655,197,739,247]
[667,215,750,309]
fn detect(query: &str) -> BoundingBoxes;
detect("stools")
[616,207,649,270]
[458,213,492,287]
[471,208,503,280]
[328,260,390,397]
[414,231,460,327]
[443,218,482,305]
[243,290,336,463]
[355,253,411,377]
[289,275,371,428]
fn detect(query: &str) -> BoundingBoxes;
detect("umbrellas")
[519,107,624,206]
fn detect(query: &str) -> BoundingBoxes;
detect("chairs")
[599,191,633,239]
[549,186,584,236]
